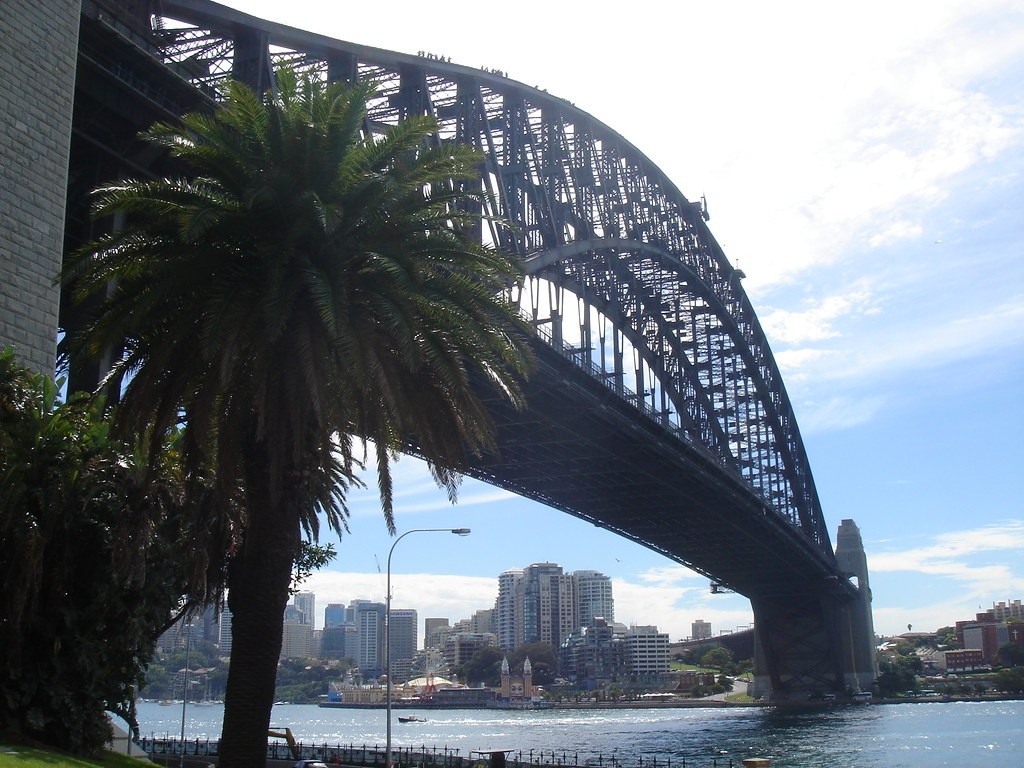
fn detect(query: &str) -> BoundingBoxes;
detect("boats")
[398,715,427,722]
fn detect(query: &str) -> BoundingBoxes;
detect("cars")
[294,759,328,768]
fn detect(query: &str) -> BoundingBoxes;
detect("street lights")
[384,528,473,768]
